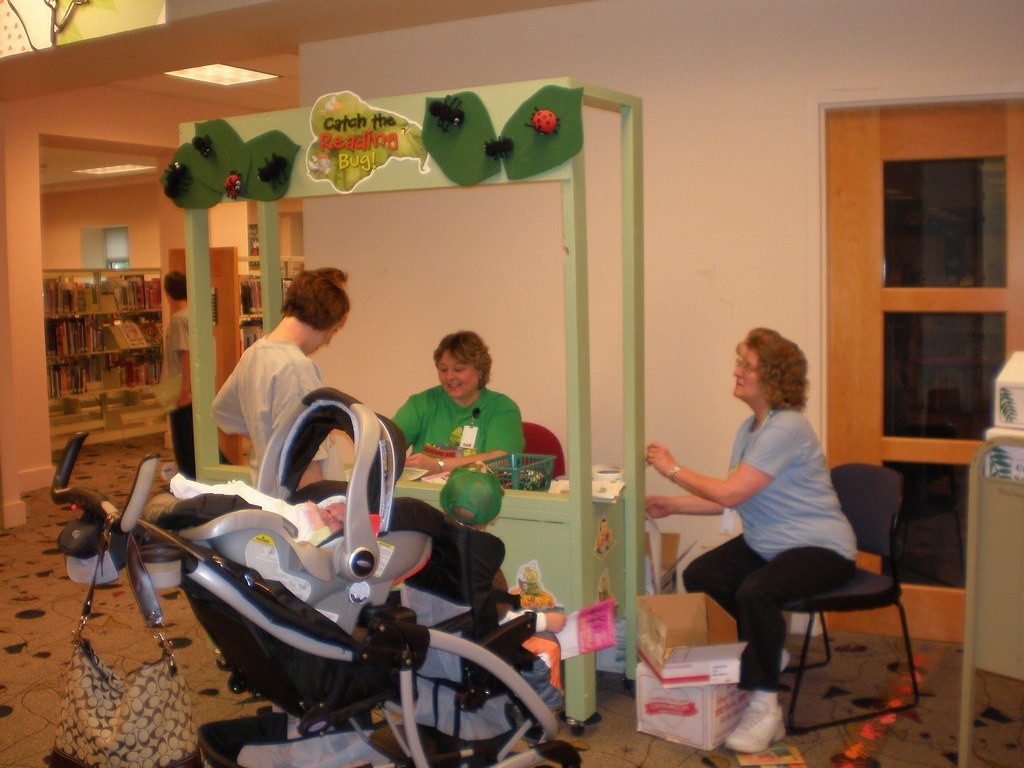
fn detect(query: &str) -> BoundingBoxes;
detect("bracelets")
[670,464,683,482]
[436,457,445,472]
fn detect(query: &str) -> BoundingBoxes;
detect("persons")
[390,331,523,478]
[438,460,590,749]
[170,473,347,546]
[165,270,197,482]
[644,328,855,754]
[211,267,350,498]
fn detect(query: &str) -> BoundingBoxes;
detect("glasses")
[736,359,759,375]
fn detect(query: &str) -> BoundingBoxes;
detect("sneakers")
[725,698,786,753]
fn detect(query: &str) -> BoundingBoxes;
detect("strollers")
[51,388,584,767]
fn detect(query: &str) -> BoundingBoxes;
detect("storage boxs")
[636,533,750,751]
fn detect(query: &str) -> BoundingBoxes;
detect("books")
[43,278,163,400]
[241,277,292,357]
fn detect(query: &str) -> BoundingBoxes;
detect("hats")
[440,461,504,525]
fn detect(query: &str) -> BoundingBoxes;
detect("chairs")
[768,463,918,733]
[883,422,965,579]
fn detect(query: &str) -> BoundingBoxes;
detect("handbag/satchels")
[54,513,202,768]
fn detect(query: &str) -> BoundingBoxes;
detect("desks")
[201,465,637,726]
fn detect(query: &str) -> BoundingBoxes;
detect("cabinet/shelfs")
[41,247,304,465]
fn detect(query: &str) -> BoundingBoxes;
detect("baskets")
[485,452,556,492]
